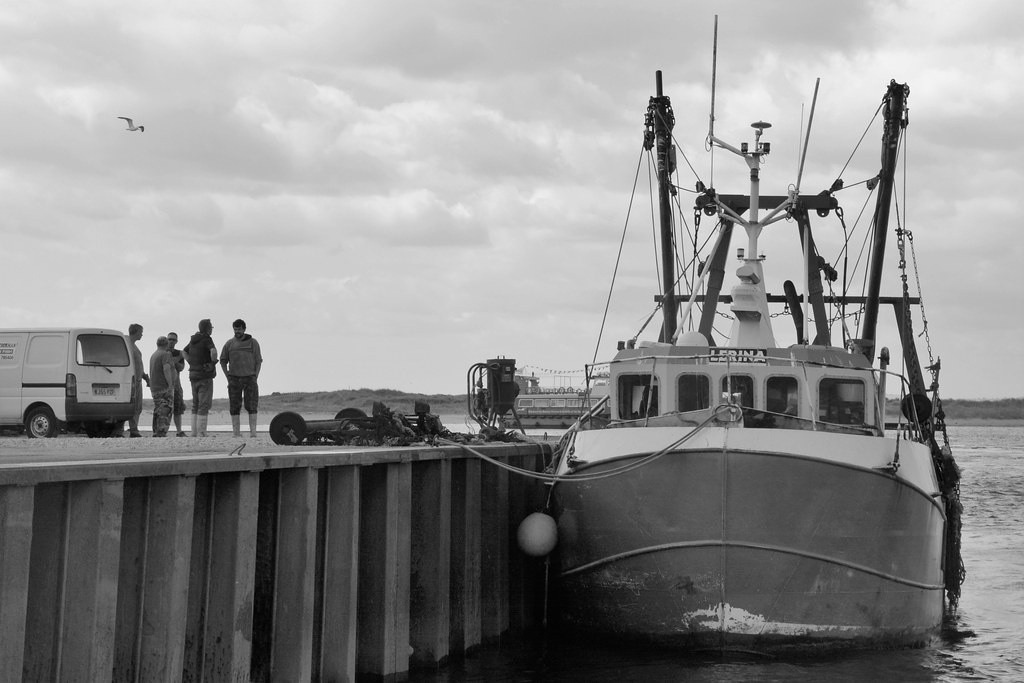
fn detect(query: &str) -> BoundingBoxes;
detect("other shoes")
[129,433,143,437]
[176,430,188,437]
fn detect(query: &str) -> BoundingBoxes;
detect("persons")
[166,333,187,437]
[113,324,150,438]
[150,336,174,437]
[182,319,217,437]
[220,320,262,438]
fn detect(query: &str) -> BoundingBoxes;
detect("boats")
[532,11,949,654]
[496,372,611,431]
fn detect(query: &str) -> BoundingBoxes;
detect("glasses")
[168,339,177,341]
[233,328,244,331]
[211,326,214,329]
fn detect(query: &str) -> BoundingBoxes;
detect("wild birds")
[118,116,144,132]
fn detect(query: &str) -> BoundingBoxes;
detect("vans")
[0,324,143,440]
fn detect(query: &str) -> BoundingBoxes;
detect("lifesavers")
[558,387,565,394]
[567,387,573,393]
